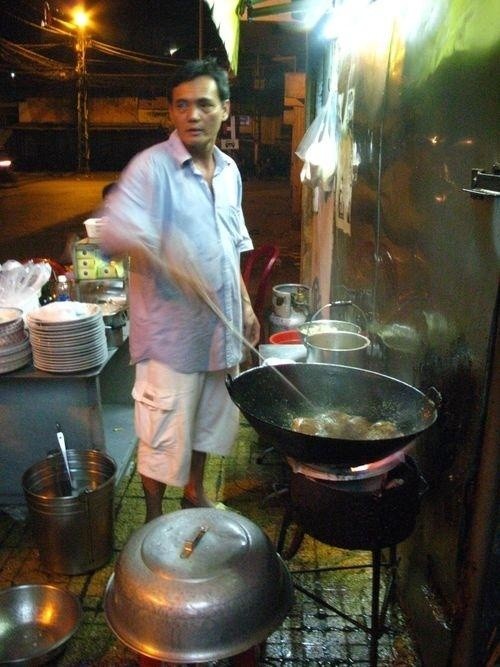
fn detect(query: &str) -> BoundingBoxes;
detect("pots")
[224,361,444,469]
[301,332,371,369]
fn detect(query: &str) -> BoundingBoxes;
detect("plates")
[0,299,109,374]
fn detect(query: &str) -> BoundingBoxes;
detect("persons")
[96,58,263,525]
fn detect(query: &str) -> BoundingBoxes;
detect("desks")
[0,321,136,506]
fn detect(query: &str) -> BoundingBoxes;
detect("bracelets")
[242,295,251,303]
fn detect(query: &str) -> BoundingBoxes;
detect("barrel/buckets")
[376,297,436,389]
[302,300,374,370]
[20,447,118,577]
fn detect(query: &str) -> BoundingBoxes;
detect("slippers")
[182,496,243,515]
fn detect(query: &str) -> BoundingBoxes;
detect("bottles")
[55,275,71,301]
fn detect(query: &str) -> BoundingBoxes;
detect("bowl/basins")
[100,505,296,666]
[0,584,85,667]
[269,318,363,345]
